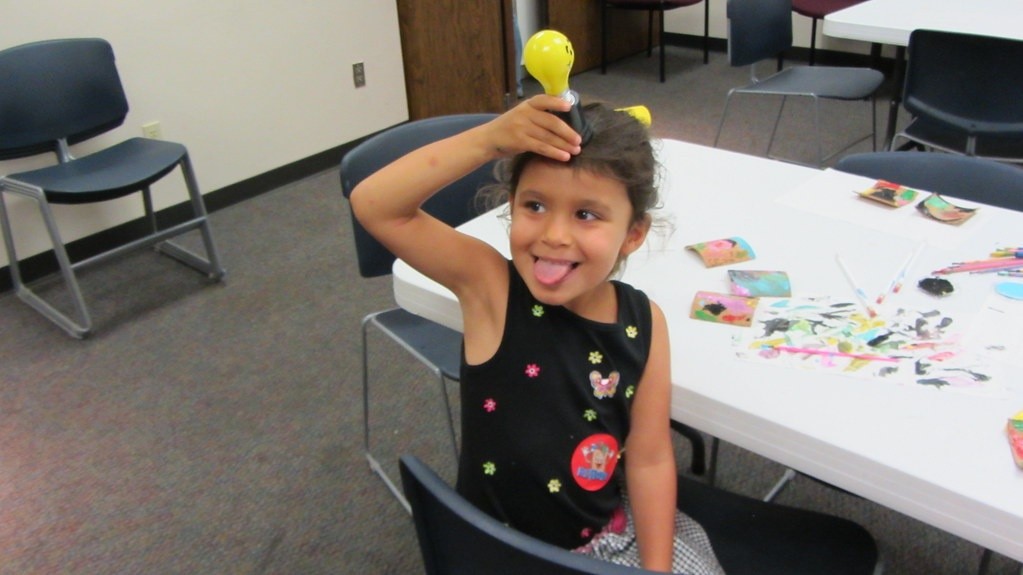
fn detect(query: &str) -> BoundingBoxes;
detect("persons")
[349,93,877,575]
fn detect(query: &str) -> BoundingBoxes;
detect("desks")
[822,0,1023,151]
[393,139,1023,566]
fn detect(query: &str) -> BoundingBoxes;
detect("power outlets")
[352,62,366,88]
[143,121,165,141]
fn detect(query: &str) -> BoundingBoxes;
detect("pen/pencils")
[931,245,1023,278]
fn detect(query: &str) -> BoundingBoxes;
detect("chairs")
[889,29,1023,170]
[339,114,503,519]
[711,0,884,171]
[398,453,885,575]
[597,0,710,84]
[0,37,227,341]
[710,152,1023,575]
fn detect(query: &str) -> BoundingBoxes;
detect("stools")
[775,0,906,102]
